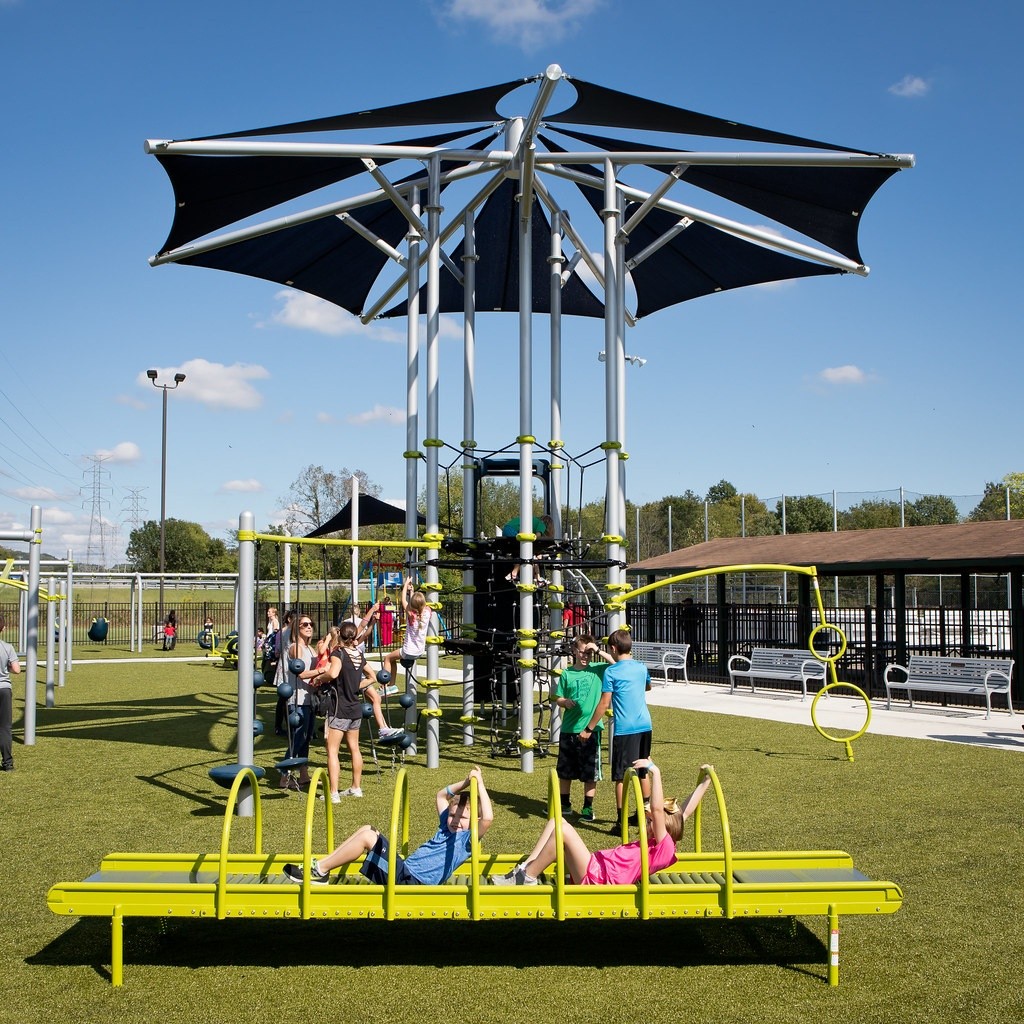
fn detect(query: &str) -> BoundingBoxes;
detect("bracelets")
[585,726,593,733]
[646,762,654,770]
[446,783,455,798]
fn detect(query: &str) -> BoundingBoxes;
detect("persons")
[0,614,21,771]
[678,598,705,667]
[162,575,432,803]
[550,597,653,838]
[282,766,495,885]
[491,756,714,887]
[503,514,555,589]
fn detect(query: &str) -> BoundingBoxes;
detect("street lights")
[146,368,187,627]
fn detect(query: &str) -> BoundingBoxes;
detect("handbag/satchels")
[314,684,332,717]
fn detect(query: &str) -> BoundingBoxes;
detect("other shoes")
[611,823,630,838]
[340,787,363,798]
[628,811,638,826]
[542,801,572,815]
[319,792,341,803]
[280,776,302,788]
[580,806,595,821]
[3,765,14,771]
[299,772,322,785]
[377,686,399,698]
[274,726,281,736]
[378,726,404,738]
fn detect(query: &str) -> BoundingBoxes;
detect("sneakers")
[283,858,329,885]
[492,862,537,885]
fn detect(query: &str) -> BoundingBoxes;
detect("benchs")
[631,641,690,687]
[727,647,830,702]
[883,654,1015,719]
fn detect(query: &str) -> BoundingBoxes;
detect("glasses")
[299,622,314,627]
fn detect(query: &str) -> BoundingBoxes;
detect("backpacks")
[263,626,282,661]
